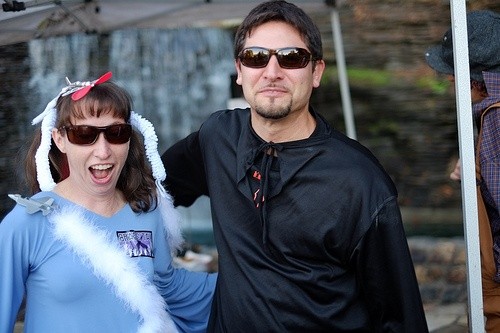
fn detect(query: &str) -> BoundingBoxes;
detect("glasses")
[59,123,132,145]
[236,47,316,69]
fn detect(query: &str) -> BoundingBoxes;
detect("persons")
[160,0,432,332]
[422,7,500,333]
[0,71,221,332]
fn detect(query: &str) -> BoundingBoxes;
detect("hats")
[425,8,500,75]
